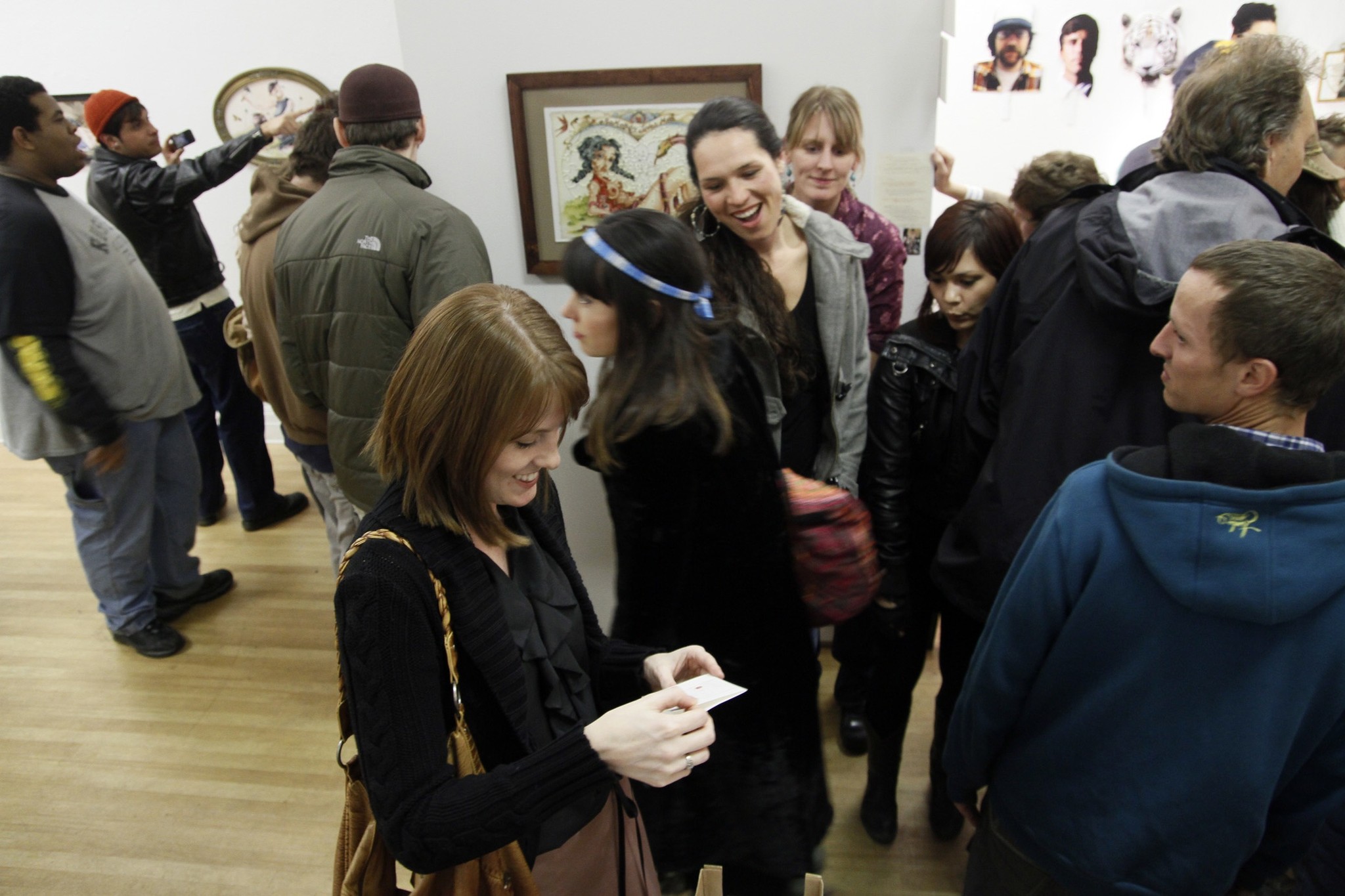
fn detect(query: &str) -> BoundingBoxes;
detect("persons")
[557,208,833,895]
[1,73,239,649]
[676,93,874,697]
[777,84,907,643]
[235,90,357,580]
[273,62,498,573]
[327,283,761,894]
[82,86,312,533]
[860,0,1345,896]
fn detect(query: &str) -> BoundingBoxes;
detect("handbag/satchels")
[778,470,876,621]
[335,723,538,896]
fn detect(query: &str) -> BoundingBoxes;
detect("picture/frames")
[503,61,767,278]
[49,94,108,164]
[212,66,335,172]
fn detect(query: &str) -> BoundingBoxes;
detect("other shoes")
[929,780,966,840]
[862,780,895,840]
[842,713,866,752]
[198,491,229,526]
[243,492,310,532]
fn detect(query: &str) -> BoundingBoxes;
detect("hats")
[1300,126,1345,183]
[333,63,425,124]
[84,90,139,136]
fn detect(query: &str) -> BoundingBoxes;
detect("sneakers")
[114,619,180,657]
[155,569,233,621]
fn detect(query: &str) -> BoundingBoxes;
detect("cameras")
[171,130,196,150]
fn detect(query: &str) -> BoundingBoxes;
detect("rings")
[685,755,693,770]
